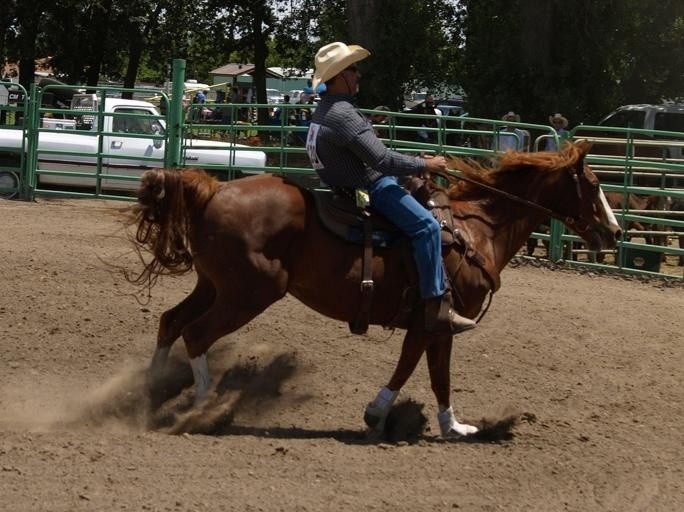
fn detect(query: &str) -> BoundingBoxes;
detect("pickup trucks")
[0,97,267,200]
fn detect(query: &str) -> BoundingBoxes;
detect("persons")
[305,42,476,333]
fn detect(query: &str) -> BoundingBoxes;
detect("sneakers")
[425,308,477,334]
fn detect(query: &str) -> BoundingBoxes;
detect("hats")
[311,41,371,90]
[502,112,520,122]
[549,113,568,129]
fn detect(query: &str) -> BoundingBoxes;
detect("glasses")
[345,65,358,74]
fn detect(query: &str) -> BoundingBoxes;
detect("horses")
[94,137,623,443]
[441,108,478,145]
[525,133,684,268]
[379,101,438,141]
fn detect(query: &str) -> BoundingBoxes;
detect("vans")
[584,104,684,188]
